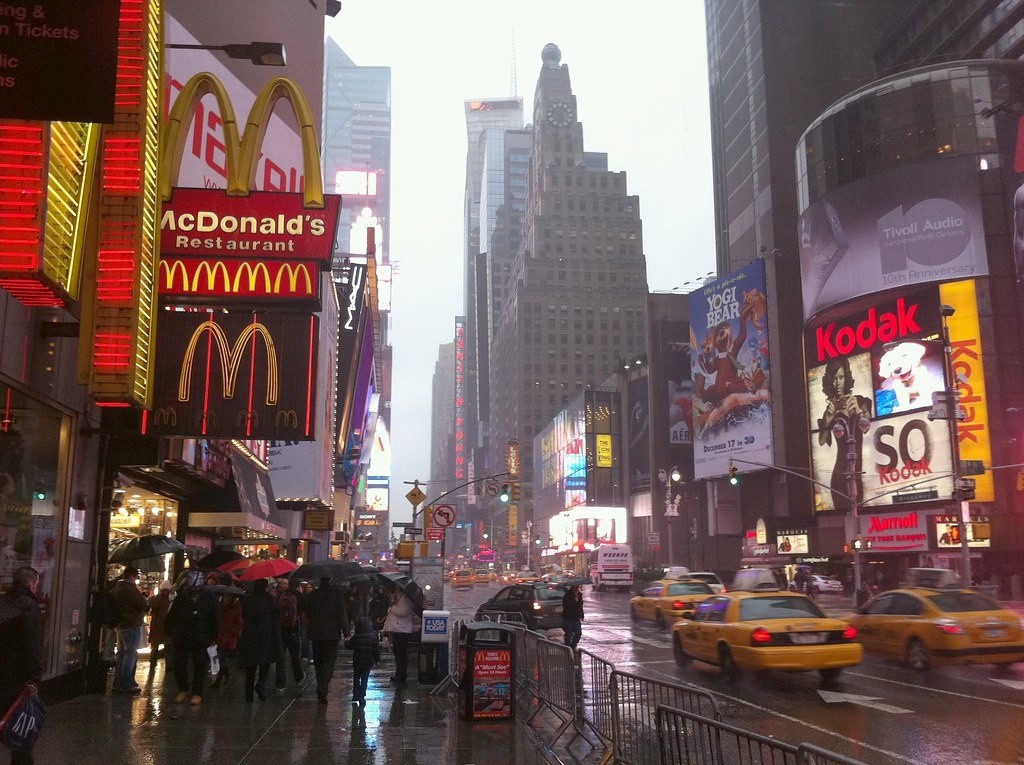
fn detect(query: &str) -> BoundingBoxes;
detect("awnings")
[186,451,287,542]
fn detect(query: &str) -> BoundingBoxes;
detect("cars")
[836,586,1023,672]
[676,572,726,594]
[451,569,474,589]
[444,570,454,581]
[474,568,490,584]
[813,575,845,594]
[629,579,715,630]
[491,568,582,592]
[673,591,865,683]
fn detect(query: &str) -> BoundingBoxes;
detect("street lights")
[832,408,873,612]
[937,303,974,592]
[659,466,684,567]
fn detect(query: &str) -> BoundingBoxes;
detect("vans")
[734,563,817,600]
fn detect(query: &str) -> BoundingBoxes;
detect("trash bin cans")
[856,589,871,614]
[459,622,518,721]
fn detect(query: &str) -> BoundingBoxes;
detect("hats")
[221,572,231,585]
[206,572,219,582]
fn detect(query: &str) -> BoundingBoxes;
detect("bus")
[591,543,635,591]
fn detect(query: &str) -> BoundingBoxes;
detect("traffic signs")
[952,491,977,501]
[405,528,422,536]
[955,478,976,488]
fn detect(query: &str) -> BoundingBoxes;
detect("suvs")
[474,584,567,635]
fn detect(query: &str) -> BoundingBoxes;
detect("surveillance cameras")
[113,492,125,507]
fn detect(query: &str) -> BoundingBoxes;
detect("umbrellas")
[556,578,593,587]
[795,565,811,572]
[108,535,187,573]
[195,549,380,581]
[376,571,424,623]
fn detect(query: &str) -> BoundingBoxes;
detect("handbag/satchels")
[0,685,48,761]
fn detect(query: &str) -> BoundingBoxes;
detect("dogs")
[879,342,934,409]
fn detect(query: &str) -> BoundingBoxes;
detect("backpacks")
[279,593,297,628]
[105,591,120,630]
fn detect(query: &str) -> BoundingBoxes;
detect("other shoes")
[256,686,265,701]
[276,686,285,692]
[317,687,327,704]
[245,689,253,703]
[111,684,141,693]
[190,694,200,704]
[391,676,406,681]
[352,692,365,702]
[294,672,307,687]
[174,691,187,703]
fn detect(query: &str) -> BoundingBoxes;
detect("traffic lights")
[500,482,510,504]
[535,535,541,546]
[729,464,738,487]
[842,543,852,553]
[865,539,873,549]
[852,538,864,551]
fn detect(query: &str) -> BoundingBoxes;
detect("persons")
[921,555,934,568]
[105,566,148,693]
[634,567,665,580]
[779,565,789,588]
[819,360,873,510]
[383,587,414,681]
[562,585,584,650]
[146,566,390,700]
[939,524,956,544]
[0,567,42,765]
[779,537,791,552]
[794,569,815,600]
